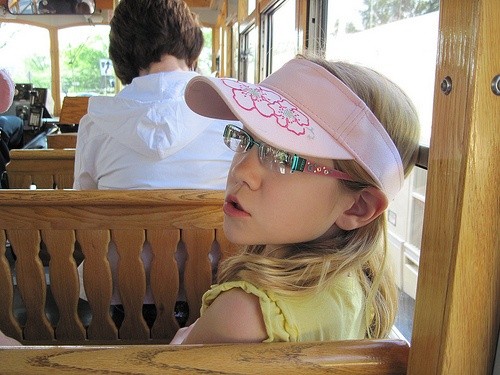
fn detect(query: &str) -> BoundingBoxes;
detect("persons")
[151,53,422,365]
[0,113,35,138]
[70,0,273,308]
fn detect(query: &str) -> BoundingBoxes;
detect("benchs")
[1,126,411,374]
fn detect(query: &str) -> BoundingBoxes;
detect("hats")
[182,56,403,208]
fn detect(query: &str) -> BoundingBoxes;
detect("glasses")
[223,123,369,186]
